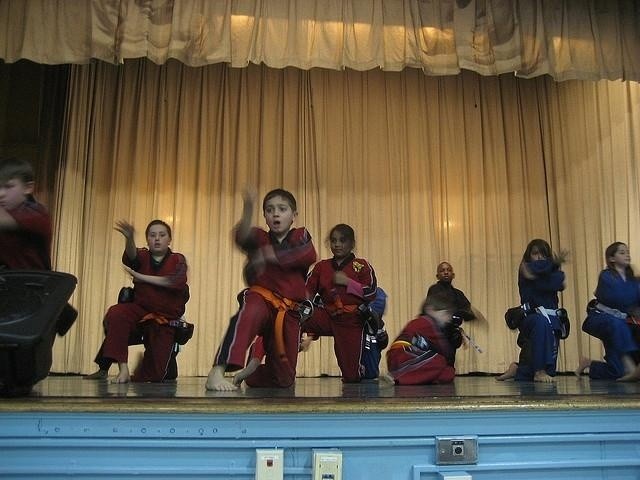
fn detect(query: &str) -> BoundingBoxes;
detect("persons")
[205,190,389,391]
[385,262,477,386]
[575,242,640,381]
[0,155,53,391]
[495,237,572,382]
[82,218,194,383]
